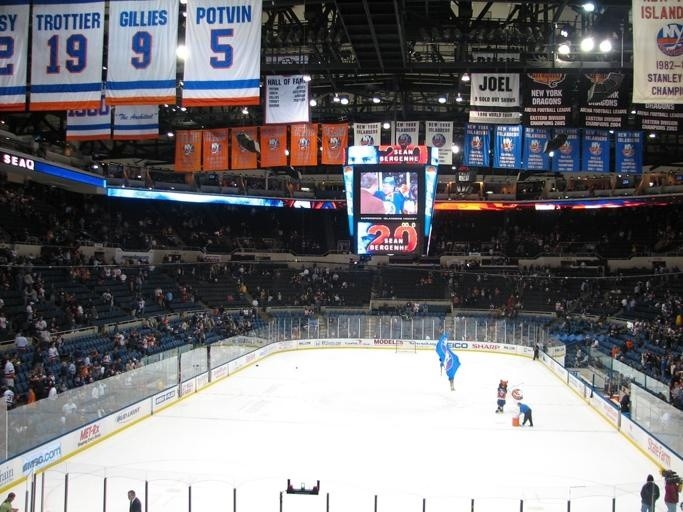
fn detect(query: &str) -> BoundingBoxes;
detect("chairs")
[0,262,683,409]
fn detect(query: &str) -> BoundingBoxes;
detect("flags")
[28,0,102,111]
[66,82,112,141]
[575,68,634,131]
[632,103,682,134]
[519,72,574,128]
[174,118,644,176]
[468,72,519,125]
[183,0,262,107]
[263,74,311,125]
[436,333,445,375]
[110,104,160,140]
[0,0,30,110]
[104,0,181,105]
[630,0,682,104]
[445,344,460,390]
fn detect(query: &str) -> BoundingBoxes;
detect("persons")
[680,501,682,512]
[0,166,681,341]
[660,468,682,512]
[571,340,682,422]
[494,380,507,413]
[0,492,18,512]
[126,491,143,512]
[640,474,659,512]
[360,172,418,214]
[540,344,546,361]
[532,343,539,360]
[0,341,257,457]
[515,401,534,426]
